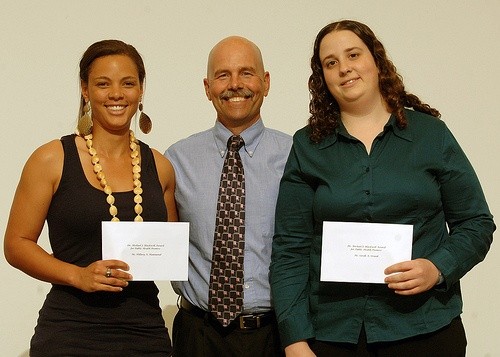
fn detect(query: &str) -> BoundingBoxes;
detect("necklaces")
[84,130,144,222]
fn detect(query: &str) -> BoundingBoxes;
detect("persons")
[163,36,294,357]
[269,21,496,357]
[4,39,178,357]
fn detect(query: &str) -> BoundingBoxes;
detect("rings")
[107,268,111,276]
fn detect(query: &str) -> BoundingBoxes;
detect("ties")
[206,134,246,330]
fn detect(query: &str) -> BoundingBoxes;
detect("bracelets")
[438,272,444,285]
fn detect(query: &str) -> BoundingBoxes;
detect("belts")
[179,296,274,330]
